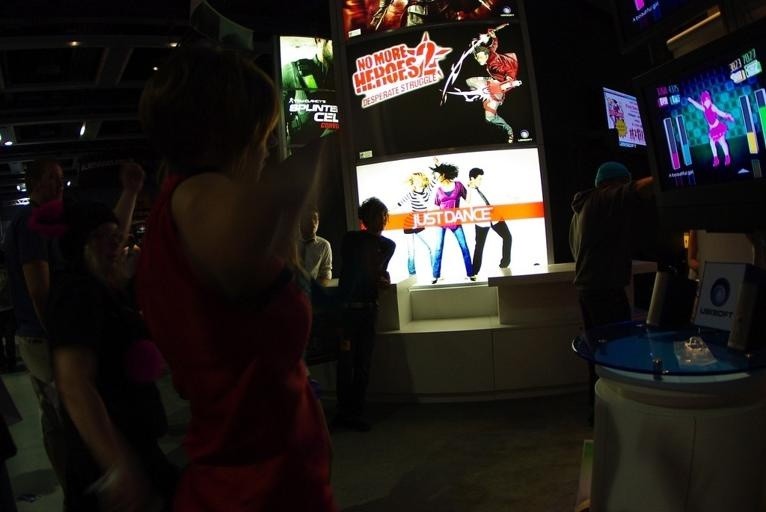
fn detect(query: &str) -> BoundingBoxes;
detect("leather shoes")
[471,276,476,281]
[432,278,438,284]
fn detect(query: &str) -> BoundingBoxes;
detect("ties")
[475,186,490,206]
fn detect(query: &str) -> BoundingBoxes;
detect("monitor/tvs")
[326,0,529,42]
[631,17,766,185]
[273,32,338,166]
[332,144,556,290]
[331,21,543,164]
[608,0,714,54]
[600,85,649,154]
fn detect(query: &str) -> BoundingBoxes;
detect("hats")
[595,161,630,187]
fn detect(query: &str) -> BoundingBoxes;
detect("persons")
[688,91,736,167]
[467,31,519,146]
[566,163,659,336]
[1,49,396,509]
[395,158,513,285]
